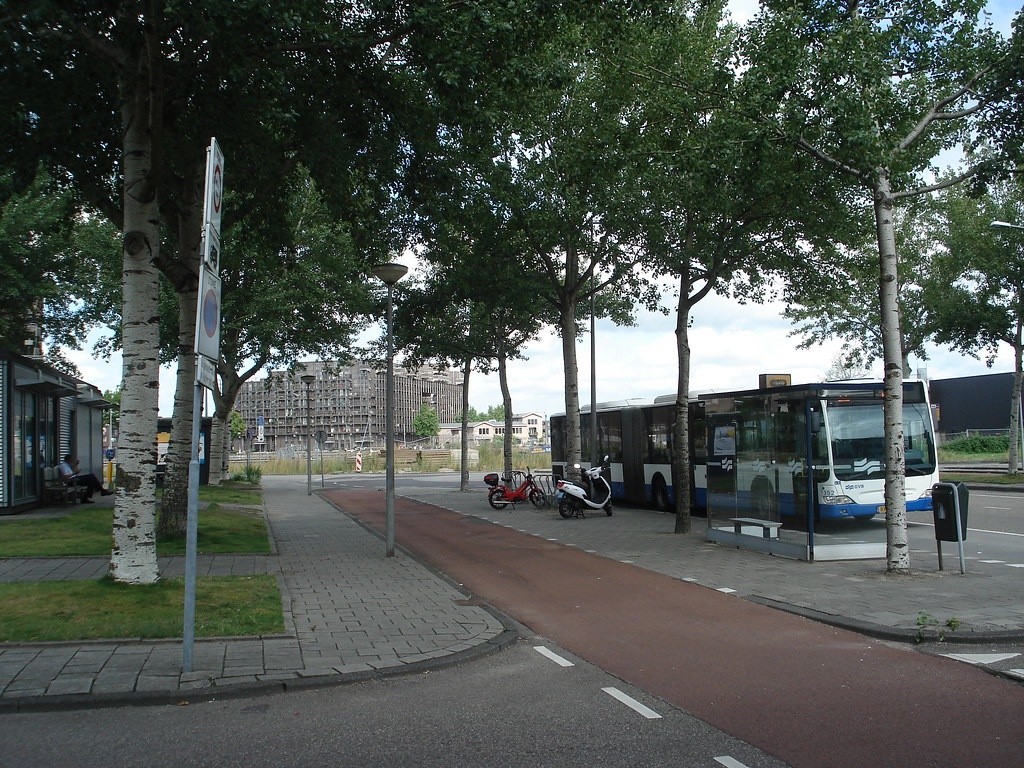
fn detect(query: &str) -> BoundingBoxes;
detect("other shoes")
[101,489,114,497]
[80,498,95,504]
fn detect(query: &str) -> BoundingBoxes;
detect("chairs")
[42,467,76,506]
[53,464,93,504]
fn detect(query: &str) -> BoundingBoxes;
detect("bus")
[549,374,942,527]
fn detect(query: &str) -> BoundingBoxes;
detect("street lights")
[301,374,317,495]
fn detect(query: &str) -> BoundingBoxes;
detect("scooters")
[480,466,545,510]
[552,456,615,517]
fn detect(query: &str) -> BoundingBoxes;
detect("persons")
[59,453,115,504]
